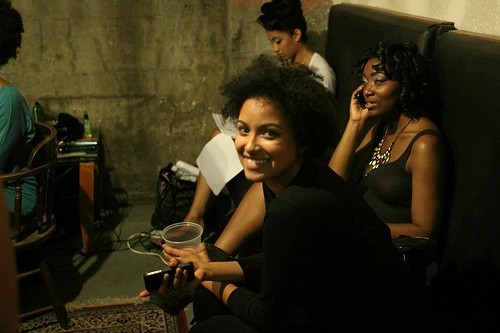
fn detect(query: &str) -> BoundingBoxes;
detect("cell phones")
[143,261,196,292]
[357,89,366,109]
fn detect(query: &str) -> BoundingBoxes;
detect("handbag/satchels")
[152,171,196,228]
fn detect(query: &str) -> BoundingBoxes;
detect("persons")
[0,0,39,232]
[327,38,443,286]
[139,54,409,332]
[177,0,336,241]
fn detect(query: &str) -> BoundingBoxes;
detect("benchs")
[419,27,500,333]
[323,1,458,142]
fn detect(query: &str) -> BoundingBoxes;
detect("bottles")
[33,102,44,122]
[84,110,93,137]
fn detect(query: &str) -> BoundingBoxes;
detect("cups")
[160,222,203,253]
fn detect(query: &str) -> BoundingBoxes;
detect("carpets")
[19,293,190,332]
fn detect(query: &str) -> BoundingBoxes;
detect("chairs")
[0,102,71,329]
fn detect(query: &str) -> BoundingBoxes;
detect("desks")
[52,125,102,255]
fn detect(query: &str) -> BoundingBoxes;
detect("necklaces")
[362,111,415,175]
